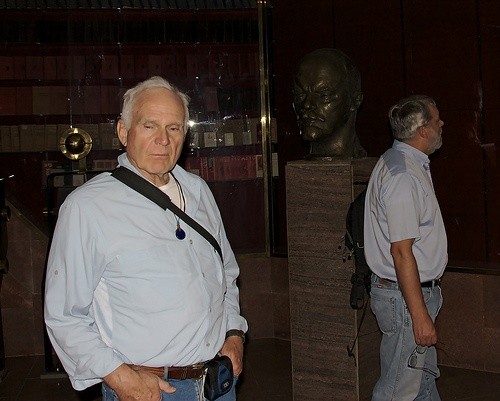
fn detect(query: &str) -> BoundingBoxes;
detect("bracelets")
[225,329,245,343]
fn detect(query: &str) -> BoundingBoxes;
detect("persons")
[291,47,368,161]
[45,76,247,401]
[363,94,449,401]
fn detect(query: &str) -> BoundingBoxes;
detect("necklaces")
[165,171,186,240]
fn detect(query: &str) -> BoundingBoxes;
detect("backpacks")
[345,187,372,307]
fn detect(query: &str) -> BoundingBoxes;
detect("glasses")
[408,340,446,376]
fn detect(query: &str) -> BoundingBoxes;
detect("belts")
[380,277,440,287]
[125,363,205,379]
